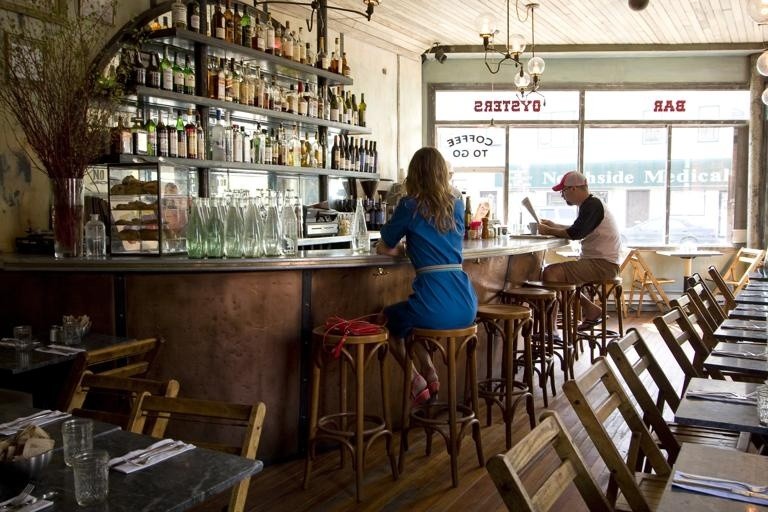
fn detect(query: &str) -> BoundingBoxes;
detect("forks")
[0,482,35,509]
[123,443,189,470]
[680,473,768,493]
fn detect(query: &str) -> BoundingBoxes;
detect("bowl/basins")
[4,447,54,478]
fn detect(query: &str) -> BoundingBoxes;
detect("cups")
[71,448,110,506]
[16,351,32,369]
[14,325,32,351]
[527,222,537,234]
[60,417,94,468]
[756,386,768,423]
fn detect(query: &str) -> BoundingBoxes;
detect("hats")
[551,171,589,190]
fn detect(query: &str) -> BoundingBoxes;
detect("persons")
[531,170,622,340]
[377,147,479,408]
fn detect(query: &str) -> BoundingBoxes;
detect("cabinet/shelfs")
[49,0,394,254]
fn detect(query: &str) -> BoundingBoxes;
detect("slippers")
[531,330,561,342]
[576,314,610,332]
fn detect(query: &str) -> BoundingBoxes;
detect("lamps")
[473,0,547,101]
[744,0,768,110]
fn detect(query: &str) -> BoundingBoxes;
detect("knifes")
[6,492,60,511]
[671,477,768,500]
[108,442,173,469]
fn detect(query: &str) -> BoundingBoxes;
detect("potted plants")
[0,0,155,261]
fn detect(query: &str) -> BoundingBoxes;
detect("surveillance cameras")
[437,50,448,64]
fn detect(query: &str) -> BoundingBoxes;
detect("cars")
[617,216,711,250]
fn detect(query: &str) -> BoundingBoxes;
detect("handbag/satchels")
[323,313,386,337]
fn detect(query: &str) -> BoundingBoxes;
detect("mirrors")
[86,5,351,243]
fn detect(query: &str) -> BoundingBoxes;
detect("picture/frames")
[4,31,49,92]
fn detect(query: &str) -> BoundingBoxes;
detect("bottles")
[350,197,370,257]
[85,214,106,259]
[169,0,351,77]
[97,107,379,173]
[101,48,369,129]
[462,190,508,240]
[186,187,298,259]
[338,193,385,231]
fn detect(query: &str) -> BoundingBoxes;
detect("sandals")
[408,372,430,407]
[427,366,439,392]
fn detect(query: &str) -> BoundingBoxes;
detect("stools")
[304,321,397,511]
[463,303,535,454]
[397,324,486,491]
